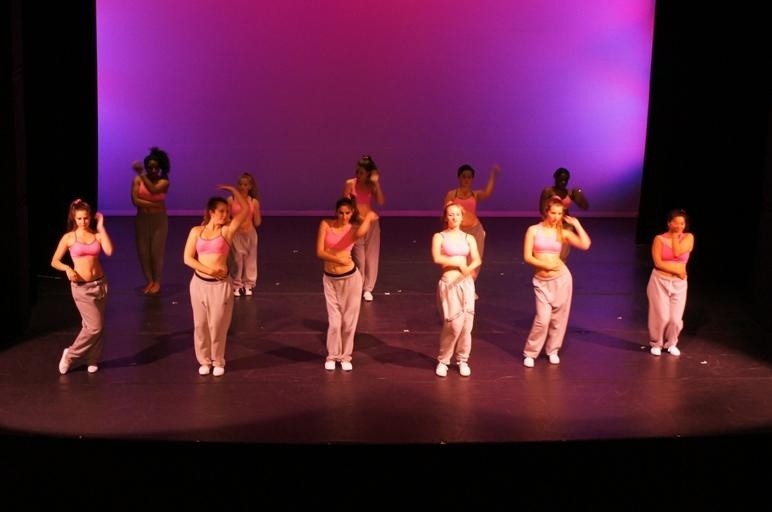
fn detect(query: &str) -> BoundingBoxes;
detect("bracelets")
[578,189,581,192]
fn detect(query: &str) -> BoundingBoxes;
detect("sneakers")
[233,288,244,296]
[142,285,150,294]
[59,349,68,375]
[667,346,680,355]
[436,363,447,376]
[363,291,373,300]
[213,367,224,376]
[199,366,209,375]
[651,347,661,355]
[325,361,335,370]
[524,358,534,367]
[244,286,253,295]
[341,362,352,371]
[88,364,98,373]
[457,362,471,376]
[150,284,160,294]
[549,355,560,364]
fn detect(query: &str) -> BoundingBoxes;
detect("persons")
[50,198,114,375]
[342,152,385,302]
[646,207,695,357]
[523,194,591,368]
[228,169,262,296]
[539,164,590,265]
[182,182,250,377]
[130,144,170,295]
[440,162,503,301]
[315,192,379,372]
[431,201,482,378]
